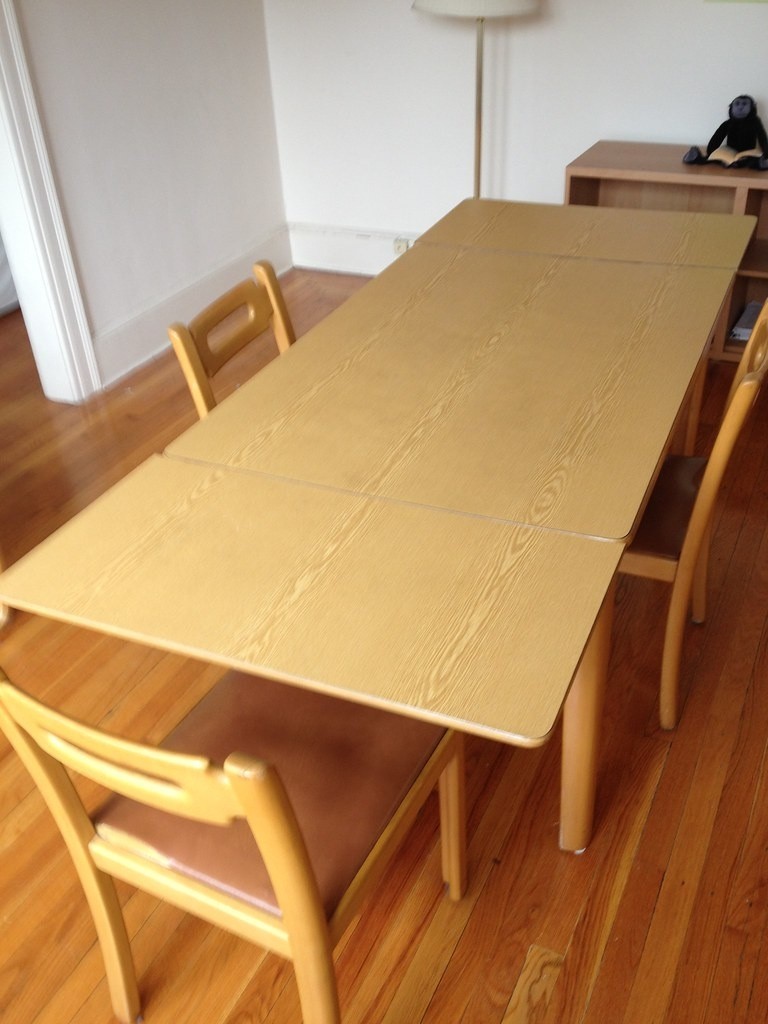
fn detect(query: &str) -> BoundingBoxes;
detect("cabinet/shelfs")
[564,139,768,362]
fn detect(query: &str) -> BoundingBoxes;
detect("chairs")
[619,294,768,732]
[2,667,468,1024]
[167,257,297,419]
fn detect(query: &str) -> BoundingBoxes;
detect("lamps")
[409,1,536,199]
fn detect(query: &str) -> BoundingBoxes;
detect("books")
[707,145,763,167]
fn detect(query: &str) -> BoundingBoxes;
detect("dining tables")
[0,198,759,856]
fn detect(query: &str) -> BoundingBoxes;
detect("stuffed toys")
[682,95,768,170]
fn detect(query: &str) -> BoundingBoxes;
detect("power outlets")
[393,238,408,253]
